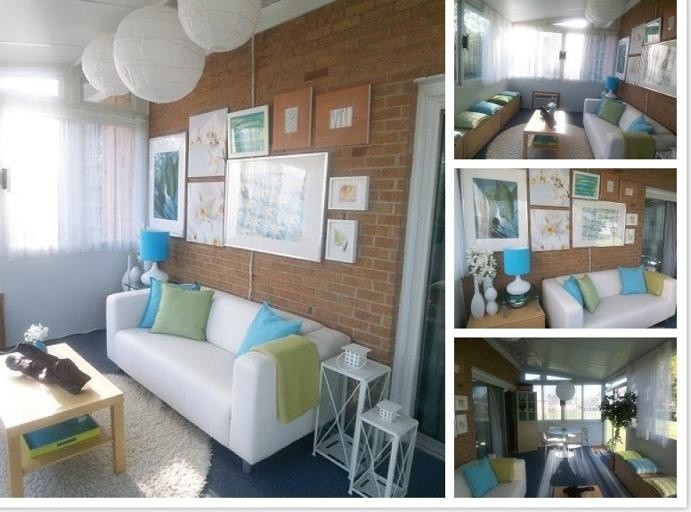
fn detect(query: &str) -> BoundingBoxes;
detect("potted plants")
[598,390,639,472]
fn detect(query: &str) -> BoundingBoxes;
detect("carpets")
[0,371,214,497]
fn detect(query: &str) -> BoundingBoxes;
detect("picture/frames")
[620,179,640,206]
[626,213,638,226]
[271,87,311,149]
[455,415,457,437]
[456,169,529,252]
[226,104,269,158]
[613,35,630,81]
[458,414,469,434]
[640,16,662,44]
[314,83,372,147]
[629,22,644,55]
[601,171,620,201]
[660,2,677,41]
[223,152,328,263]
[185,181,226,249]
[638,40,677,98]
[624,228,635,246]
[625,56,643,86]
[530,209,570,252]
[324,218,357,267]
[455,394,469,411]
[186,107,226,178]
[146,130,187,238]
[571,199,626,248]
[527,169,570,208]
[571,170,601,200]
[328,175,368,213]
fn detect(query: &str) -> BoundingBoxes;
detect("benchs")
[613,448,677,497]
[454,88,523,158]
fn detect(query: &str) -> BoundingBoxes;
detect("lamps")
[503,246,532,309]
[584,5,610,24]
[82,32,128,97]
[602,75,620,99]
[138,226,171,285]
[555,380,575,401]
[588,1,628,23]
[113,6,205,104]
[176,0,262,54]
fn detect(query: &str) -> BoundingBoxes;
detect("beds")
[524,109,569,159]
[548,426,582,458]
[554,483,603,498]
[0,342,126,497]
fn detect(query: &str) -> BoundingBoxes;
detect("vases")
[608,457,616,472]
[470,277,485,320]
[485,287,499,316]
[32,340,48,353]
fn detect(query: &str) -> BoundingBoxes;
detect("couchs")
[582,98,677,159]
[454,454,526,497]
[105,283,354,475]
[541,264,677,329]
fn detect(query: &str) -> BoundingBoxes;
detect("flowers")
[23,323,49,342]
[466,247,487,277]
[484,250,497,286]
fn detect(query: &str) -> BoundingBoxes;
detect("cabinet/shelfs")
[511,391,538,455]
[464,295,546,329]
[347,398,420,498]
[311,345,393,479]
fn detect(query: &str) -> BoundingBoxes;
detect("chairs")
[575,426,590,451]
[564,433,584,463]
[541,432,566,461]
